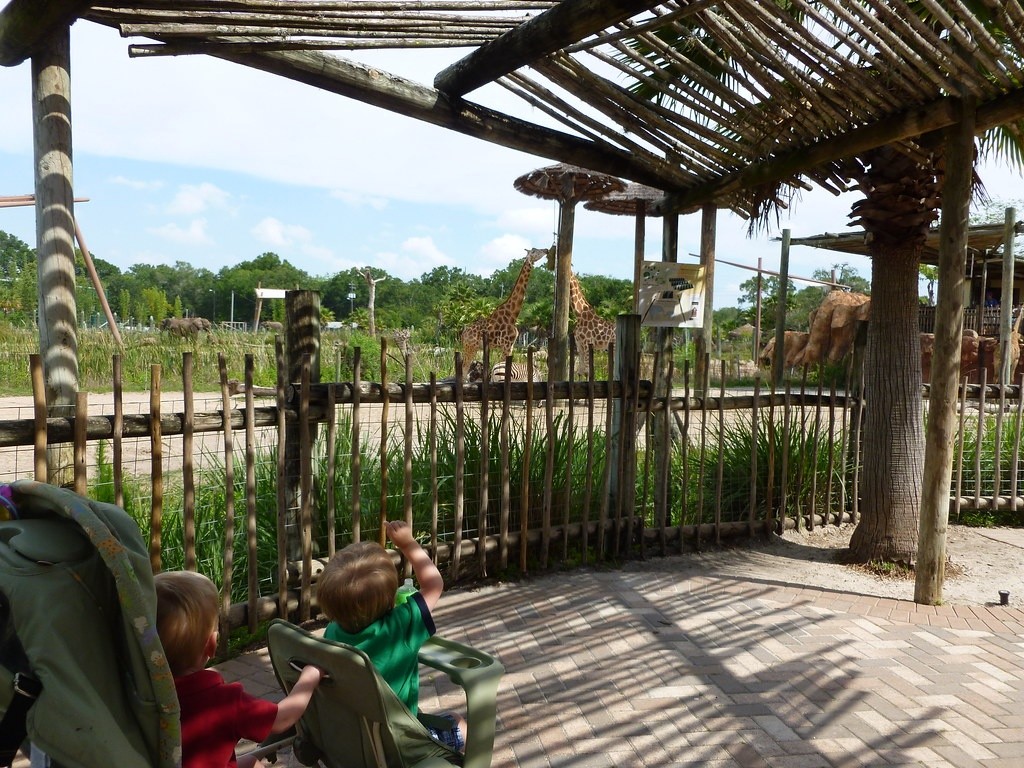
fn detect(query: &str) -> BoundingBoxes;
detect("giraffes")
[463,248,548,377]
[993,308,1024,404]
[544,244,616,405]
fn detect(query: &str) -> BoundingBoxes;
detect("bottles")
[397,578,417,609]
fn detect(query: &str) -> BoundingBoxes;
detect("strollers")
[1,478,507,768]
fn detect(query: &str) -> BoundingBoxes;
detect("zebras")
[490,360,545,409]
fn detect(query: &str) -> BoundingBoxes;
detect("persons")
[152,570,323,768]
[315,520,467,756]
